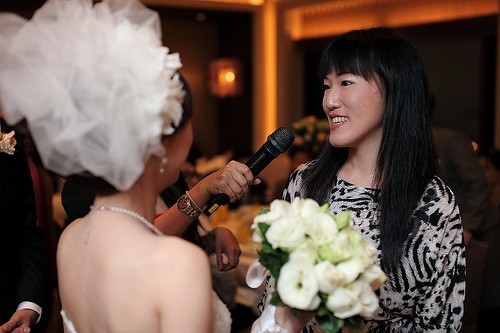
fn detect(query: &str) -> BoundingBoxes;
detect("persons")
[0,0,233,333]
[0,114,54,333]
[61,160,262,272]
[428,93,500,309]
[245,26,466,333]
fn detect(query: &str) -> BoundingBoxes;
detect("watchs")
[176,195,201,220]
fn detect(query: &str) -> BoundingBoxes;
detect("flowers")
[285,116,330,159]
[251,195,388,333]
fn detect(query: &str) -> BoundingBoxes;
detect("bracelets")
[185,190,203,212]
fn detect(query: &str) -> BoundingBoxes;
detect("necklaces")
[88,205,163,235]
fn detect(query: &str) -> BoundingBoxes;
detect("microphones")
[203,127,295,217]
